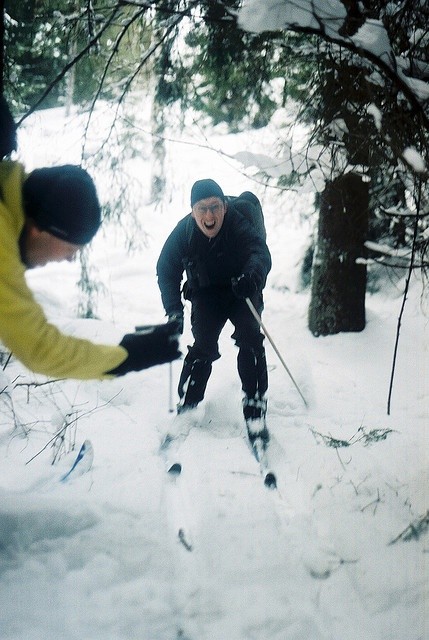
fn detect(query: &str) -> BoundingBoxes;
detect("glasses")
[197,201,223,215]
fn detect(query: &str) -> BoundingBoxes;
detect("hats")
[191,179,225,209]
[21,165,101,245]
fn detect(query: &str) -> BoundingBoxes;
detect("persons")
[0,159,183,380]
[156,178,272,442]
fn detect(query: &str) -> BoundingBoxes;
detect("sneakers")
[167,403,196,438]
[243,397,269,443]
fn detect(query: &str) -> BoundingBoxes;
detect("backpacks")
[227,191,266,249]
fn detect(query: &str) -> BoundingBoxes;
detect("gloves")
[169,313,184,335]
[232,274,256,299]
[122,327,182,371]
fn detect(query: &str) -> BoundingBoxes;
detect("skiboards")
[166,397,276,487]
